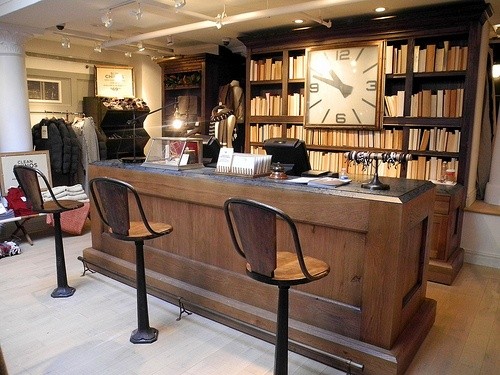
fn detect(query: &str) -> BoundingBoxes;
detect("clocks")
[302,39,387,131]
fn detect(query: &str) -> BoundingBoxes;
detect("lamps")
[174,0,186,10]
[61,34,71,49]
[124,51,131,58]
[117,89,198,165]
[93,43,103,53]
[216,12,228,30]
[98,7,114,29]
[130,1,145,21]
[136,40,146,52]
[166,34,174,47]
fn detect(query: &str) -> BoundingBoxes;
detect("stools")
[222,196,332,375]
[88,177,175,346]
[12,163,85,299]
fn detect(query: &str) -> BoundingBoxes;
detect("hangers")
[52,111,56,121]
[60,111,66,120]
[45,111,50,120]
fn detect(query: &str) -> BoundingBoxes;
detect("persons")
[208,105,236,148]
[220,80,245,123]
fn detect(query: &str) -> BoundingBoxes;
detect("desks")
[0,212,47,247]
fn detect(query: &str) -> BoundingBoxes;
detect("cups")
[445,170,455,185]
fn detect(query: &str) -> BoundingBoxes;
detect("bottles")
[341,168,348,180]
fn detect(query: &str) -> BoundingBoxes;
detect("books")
[408,127,460,152]
[250,93,283,115]
[306,150,400,178]
[249,58,282,81]
[251,123,282,142]
[250,146,267,154]
[287,124,403,151]
[382,91,405,117]
[287,92,305,116]
[407,156,459,183]
[414,41,468,72]
[289,55,304,80]
[411,88,463,118]
[385,44,407,73]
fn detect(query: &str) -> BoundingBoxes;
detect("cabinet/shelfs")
[156,53,219,167]
[236,0,497,288]
[82,95,151,161]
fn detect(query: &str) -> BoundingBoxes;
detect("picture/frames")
[93,63,137,100]
[0,149,54,197]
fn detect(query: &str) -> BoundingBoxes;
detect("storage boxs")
[141,136,205,173]
